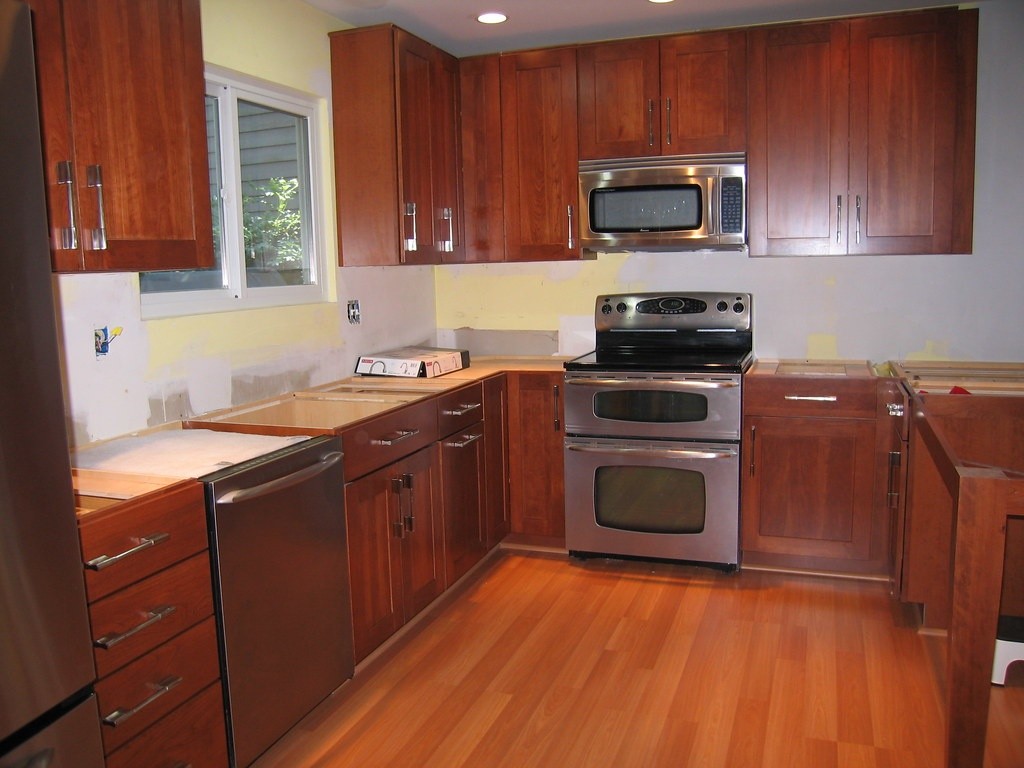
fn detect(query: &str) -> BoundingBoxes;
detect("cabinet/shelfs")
[505,369,565,537]
[746,5,978,257]
[328,23,466,267]
[437,382,488,608]
[70,466,231,768]
[576,27,747,161]
[22,0,217,275]
[892,358,1023,768]
[739,358,886,573]
[340,394,447,688]
[461,45,597,264]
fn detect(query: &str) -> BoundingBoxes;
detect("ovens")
[565,373,740,570]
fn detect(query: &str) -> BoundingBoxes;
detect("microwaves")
[578,152,746,247]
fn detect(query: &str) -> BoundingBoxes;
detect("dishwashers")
[195,433,353,768]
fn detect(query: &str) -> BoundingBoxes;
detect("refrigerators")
[0,0,108,768]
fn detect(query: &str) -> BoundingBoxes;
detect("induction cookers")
[563,291,752,373]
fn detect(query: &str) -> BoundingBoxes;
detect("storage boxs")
[355,345,472,381]
